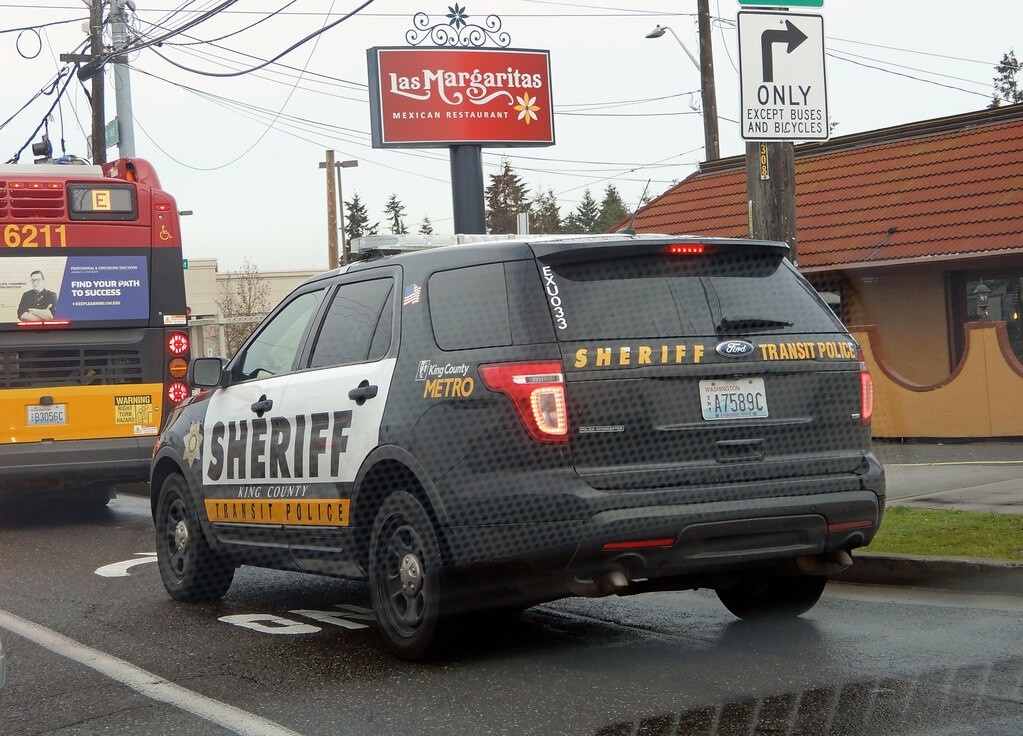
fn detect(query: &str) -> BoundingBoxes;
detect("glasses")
[30,278,43,284]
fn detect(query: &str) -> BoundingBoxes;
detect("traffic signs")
[738,11,828,143]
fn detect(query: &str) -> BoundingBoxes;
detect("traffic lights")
[32,135,53,165]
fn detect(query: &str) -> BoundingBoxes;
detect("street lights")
[319,161,358,266]
[644,24,720,162]
[973,276,991,320]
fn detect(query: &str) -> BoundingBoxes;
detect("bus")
[0,157,191,508]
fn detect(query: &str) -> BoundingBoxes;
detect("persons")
[18,271,57,322]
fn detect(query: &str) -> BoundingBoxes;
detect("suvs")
[149,237,886,659]
